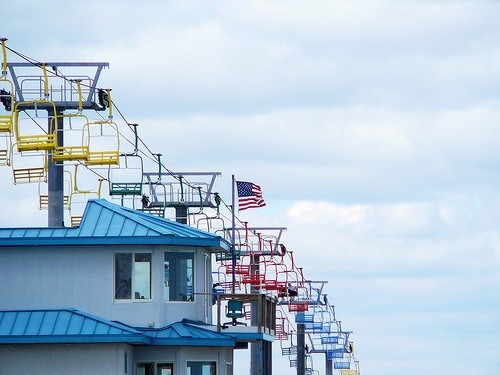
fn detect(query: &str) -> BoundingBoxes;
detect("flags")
[235,181,267,210]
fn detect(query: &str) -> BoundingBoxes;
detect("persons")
[286,283,296,293]
